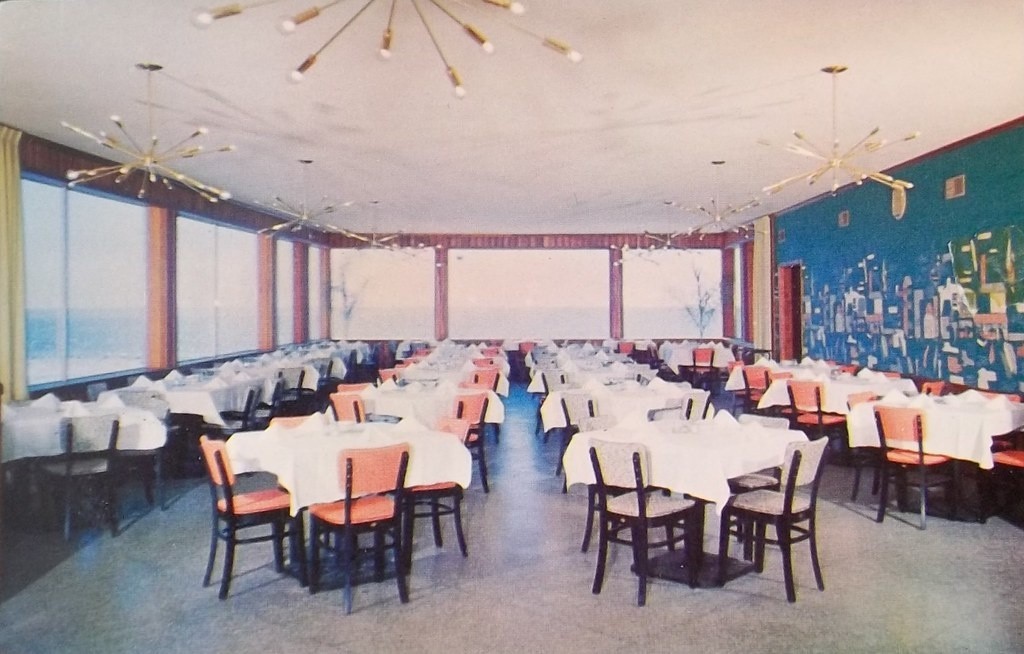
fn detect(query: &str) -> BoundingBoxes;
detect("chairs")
[0,339,1024,617]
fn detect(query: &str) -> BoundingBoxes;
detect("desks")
[227,421,471,590]
[757,379,921,412]
[847,396,1024,517]
[563,420,810,589]
[533,364,659,393]
[357,385,504,422]
[96,377,262,468]
[657,339,735,375]
[724,363,832,391]
[0,400,167,532]
[538,385,715,431]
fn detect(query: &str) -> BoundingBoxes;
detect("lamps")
[197,0,586,97]
[257,159,351,235]
[348,200,399,254]
[645,201,693,252]
[763,69,921,201]
[609,230,663,267]
[59,64,235,203]
[689,162,767,241]
